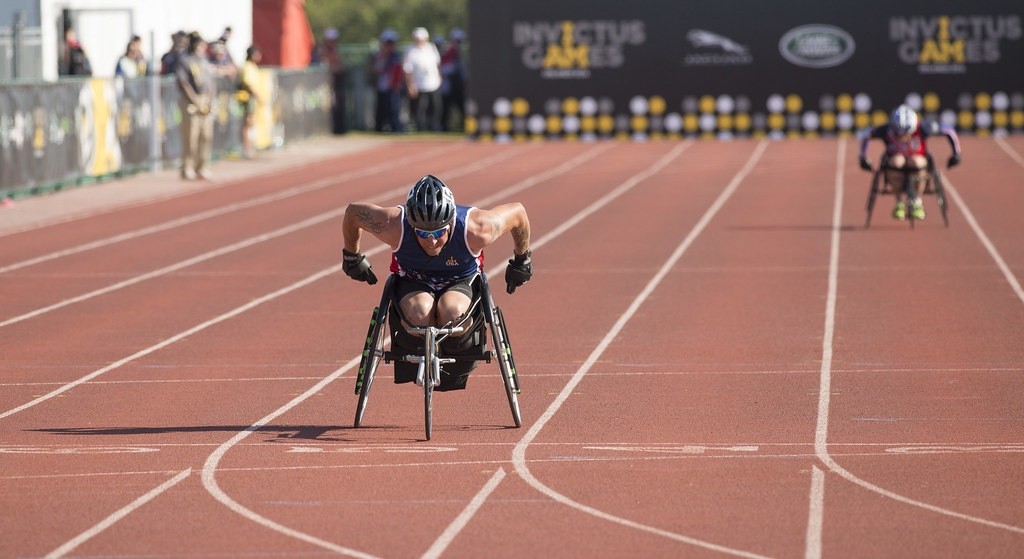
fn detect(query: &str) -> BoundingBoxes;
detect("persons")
[342,174,533,351]
[402,27,442,131]
[177,39,216,179]
[65,29,91,75]
[160,27,233,72]
[434,29,466,131]
[238,46,266,158]
[117,36,147,77]
[860,106,961,221]
[318,27,347,132]
[375,30,405,133]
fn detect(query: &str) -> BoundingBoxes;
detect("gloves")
[341,248,378,285]
[858,156,873,172]
[504,248,534,294]
[946,152,959,168]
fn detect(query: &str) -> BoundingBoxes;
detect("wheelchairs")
[864,151,951,231]
[352,270,523,440]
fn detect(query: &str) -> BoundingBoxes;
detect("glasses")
[414,224,451,239]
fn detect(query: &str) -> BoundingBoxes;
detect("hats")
[324,27,341,41]
[378,28,399,43]
[450,28,463,41]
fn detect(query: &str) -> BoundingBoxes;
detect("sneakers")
[908,196,926,220]
[891,201,907,219]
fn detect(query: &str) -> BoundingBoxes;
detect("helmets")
[404,174,455,231]
[893,104,918,135]
